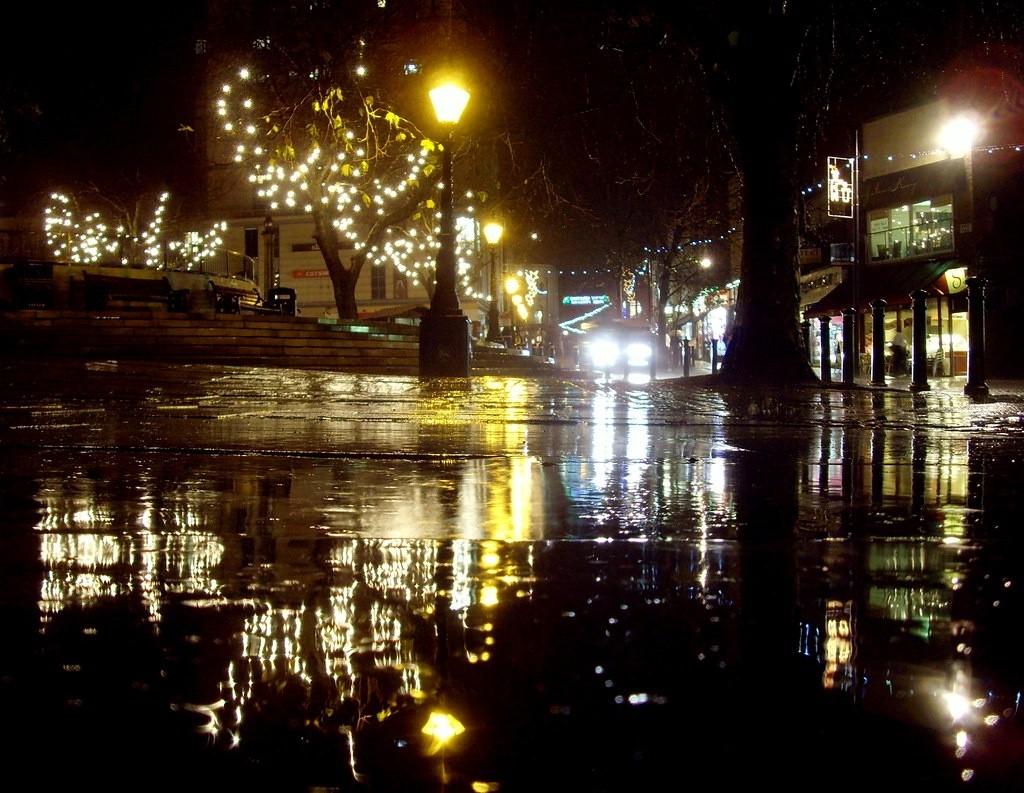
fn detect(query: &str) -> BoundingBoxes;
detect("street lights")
[482,209,504,346]
[260,215,280,298]
[513,294,529,347]
[418,35,473,379]
[504,273,517,348]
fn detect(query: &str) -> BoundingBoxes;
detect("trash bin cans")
[268,287,297,316]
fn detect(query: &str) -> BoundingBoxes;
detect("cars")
[587,326,665,376]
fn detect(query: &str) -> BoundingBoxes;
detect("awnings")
[802,253,959,320]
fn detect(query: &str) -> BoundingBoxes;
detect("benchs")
[208,280,280,314]
[81,268,179,311]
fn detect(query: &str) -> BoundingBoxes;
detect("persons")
[889,333,909,378]
